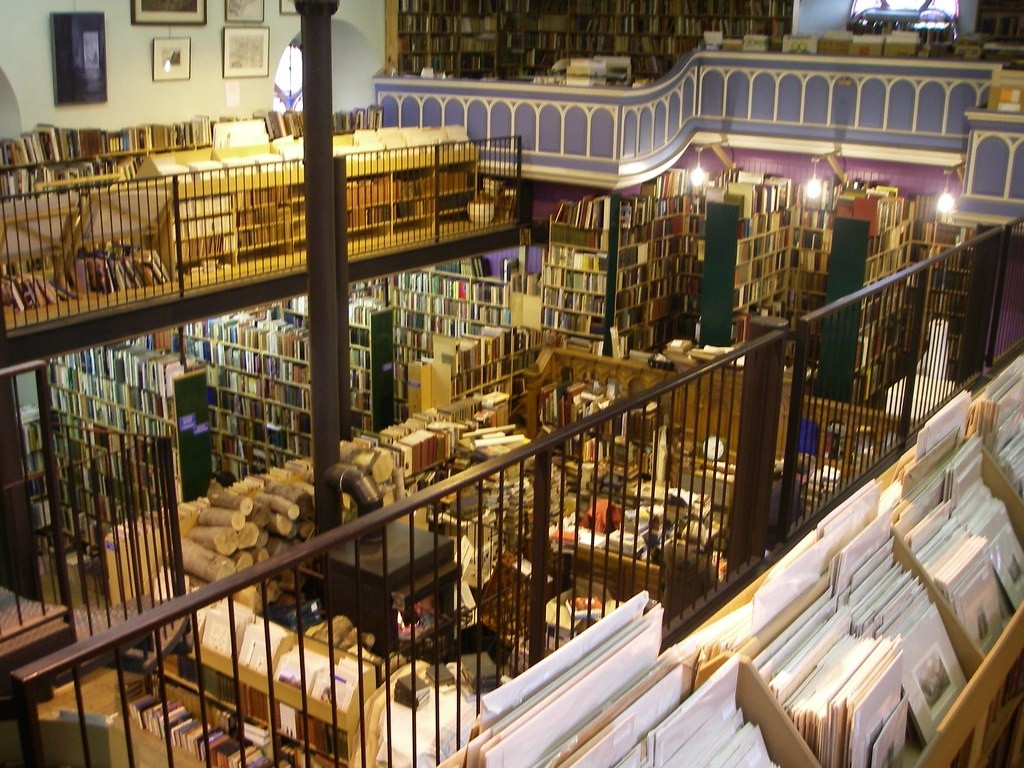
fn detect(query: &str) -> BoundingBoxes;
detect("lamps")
[691,152,704,186]
[806,162,821,198]
[937,175,955,213]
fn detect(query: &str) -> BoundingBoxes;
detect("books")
[113,374,841,768]
[396,0,1024,116]
[21,315,315,580]
[542,167,974,403]
[0,105,542,436]
[461,354,1024,768]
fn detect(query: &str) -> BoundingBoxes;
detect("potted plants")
[466,189,496,225]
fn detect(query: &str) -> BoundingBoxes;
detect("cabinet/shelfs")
[385,0,798,87]
[0,103,1023,768]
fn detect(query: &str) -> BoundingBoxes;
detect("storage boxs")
[564,37,922,88]
[986,85,1023,114]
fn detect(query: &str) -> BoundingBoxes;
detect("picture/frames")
[225,0,265,23]
[279,0,299,15]
[131,0,207,26]
[50,12,108,105]
[223,26,269,78]
[153,36,191,82]
